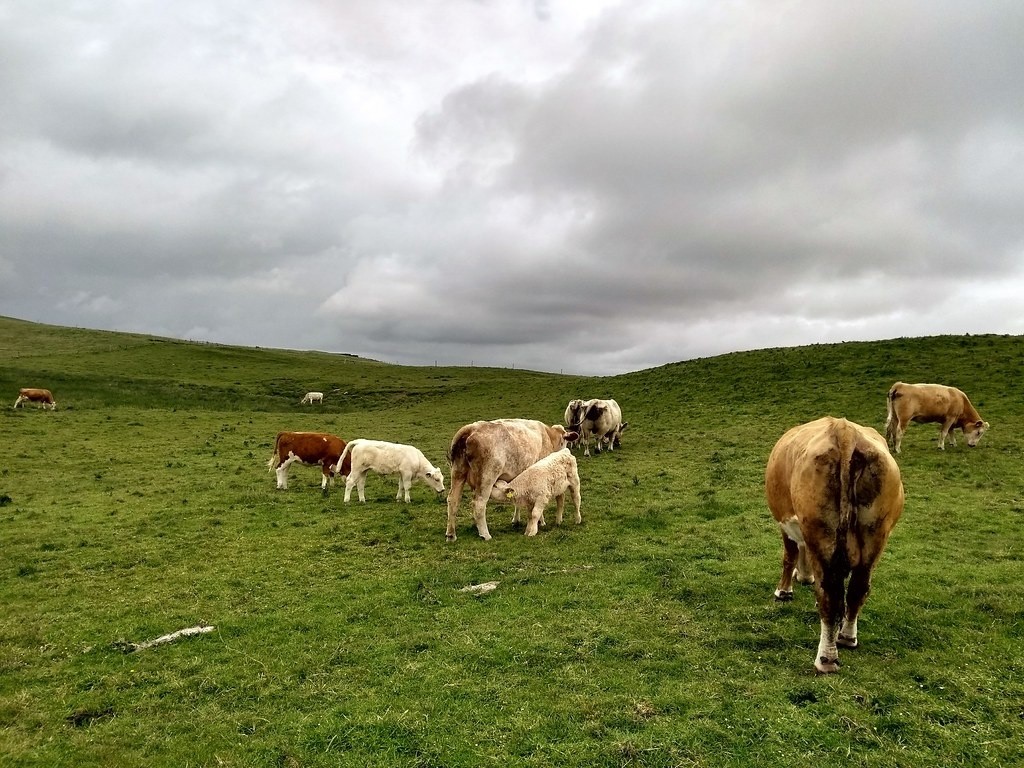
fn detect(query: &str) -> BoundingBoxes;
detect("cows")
[301,392,323,406]
[885,380,991,453]
[14,388,57,409]
[765,415,905,675]
[268,399,629,542]
